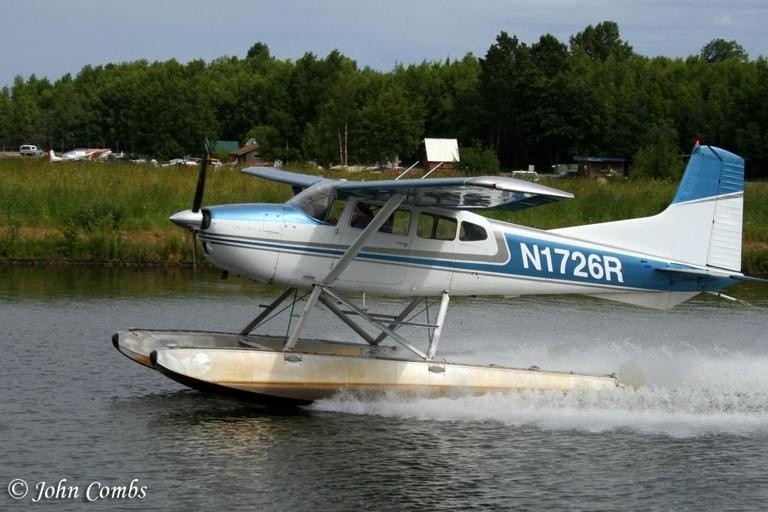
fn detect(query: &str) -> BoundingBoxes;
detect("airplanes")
[108,142,748,411]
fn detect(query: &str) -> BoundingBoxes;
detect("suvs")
[19,144,43,156]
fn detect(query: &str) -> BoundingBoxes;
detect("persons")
[328,203,374,229]
